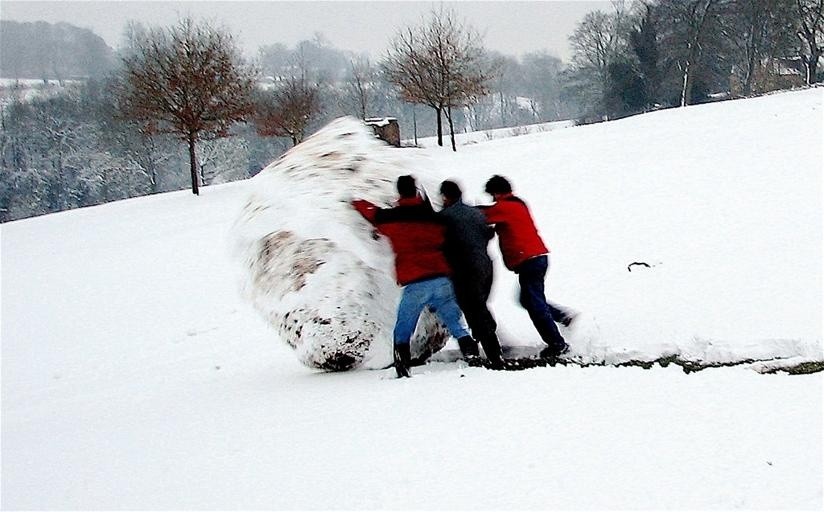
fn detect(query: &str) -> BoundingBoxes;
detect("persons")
[435,180,508,368]
[471,173,576,357]
[341,173,480,381]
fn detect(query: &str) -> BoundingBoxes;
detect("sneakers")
[540,345,569,356]
